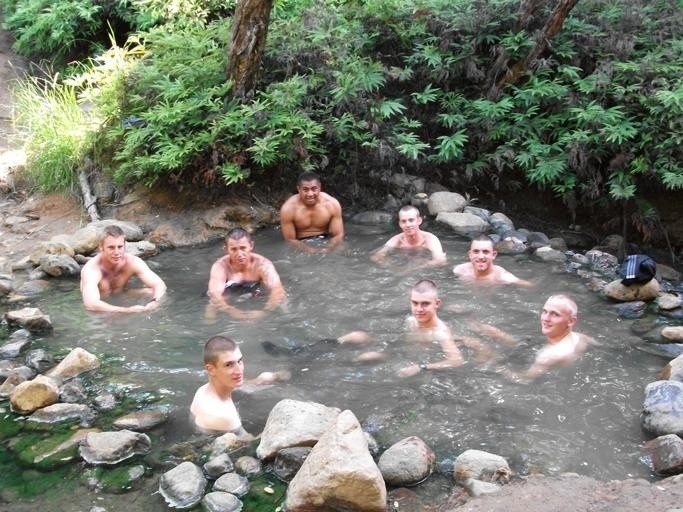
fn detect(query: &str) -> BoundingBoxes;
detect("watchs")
[418,363,427,370]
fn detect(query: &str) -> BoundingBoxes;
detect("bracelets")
[148,296,161,302]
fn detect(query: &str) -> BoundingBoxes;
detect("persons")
[278,170,345,263]
[452,292,600,389]
[199,225,291,323]
[365,203,449,275]
[77,225,168,318]
[444,232,535,291]
[258,277,465,384]
[185,333,293,443]
